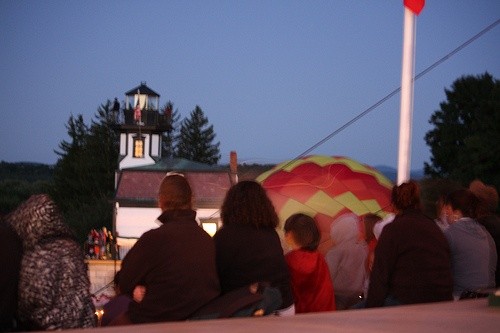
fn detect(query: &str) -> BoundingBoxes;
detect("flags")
[134,89,141,121]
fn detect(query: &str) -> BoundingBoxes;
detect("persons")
[212,181,293,317]
[323,179,500,310]
[0,185,132,333]
[100,271,133,326]
[118,175,219,325]
[283,213,337,314]
